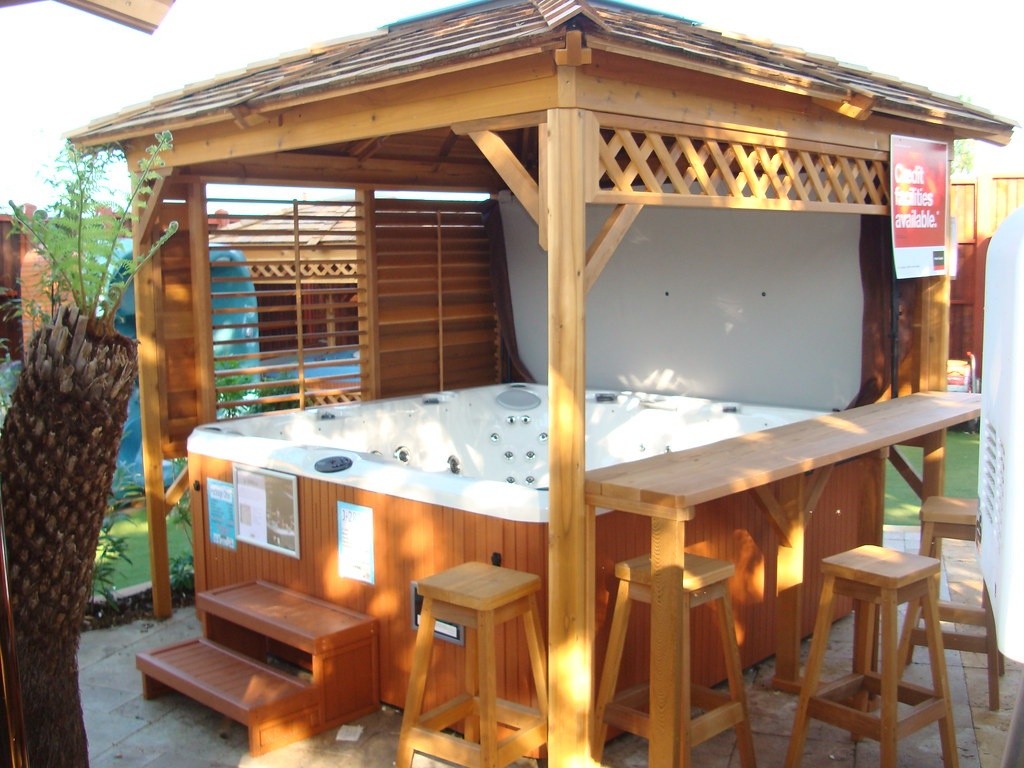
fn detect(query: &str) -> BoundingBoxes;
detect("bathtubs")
[185,382,881,748]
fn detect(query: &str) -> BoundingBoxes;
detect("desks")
[584,389,984,768]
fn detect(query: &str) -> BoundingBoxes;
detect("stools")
[898,496,1006,712]
[592,551,757,768]
[782,545,959,768]
[396,560,548,768]
